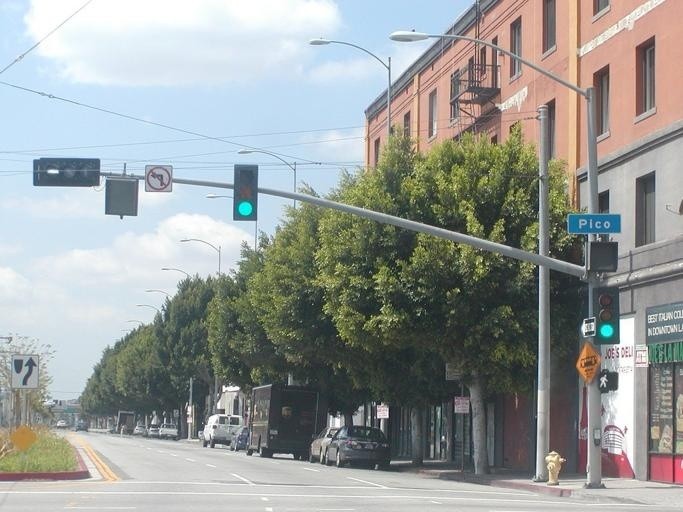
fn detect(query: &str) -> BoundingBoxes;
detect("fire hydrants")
[544,449,567,486]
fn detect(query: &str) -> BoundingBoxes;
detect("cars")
[323,424,392,468]
[142,423,158,437]
[74,422,89,432]
[306,425,338,464]
[229,424,250,452]
[56,418,69,430]
[132,424,146,436]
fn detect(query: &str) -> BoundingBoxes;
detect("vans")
[200,413,242,450]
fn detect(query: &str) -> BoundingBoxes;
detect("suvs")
[157,422,179,439]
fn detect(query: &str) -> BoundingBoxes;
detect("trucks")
[245,383,319,461]
[115,411,135,434]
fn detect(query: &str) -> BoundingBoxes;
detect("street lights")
[205,192,259,252]
[307,36,392,152]
[179,237,222,276]
[144,288,173,299]
[236,147,298,213]
[134,302,160,312]
[159,266,195,281]
[391,28,610,491]
[118,319,143,333]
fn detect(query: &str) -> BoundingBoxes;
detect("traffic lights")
[231,163,258,222]
[596,368,620,394]
[591,286,620,345]
[37,157,101,187]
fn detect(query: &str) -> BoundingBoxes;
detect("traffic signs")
[580,316,597,338]
